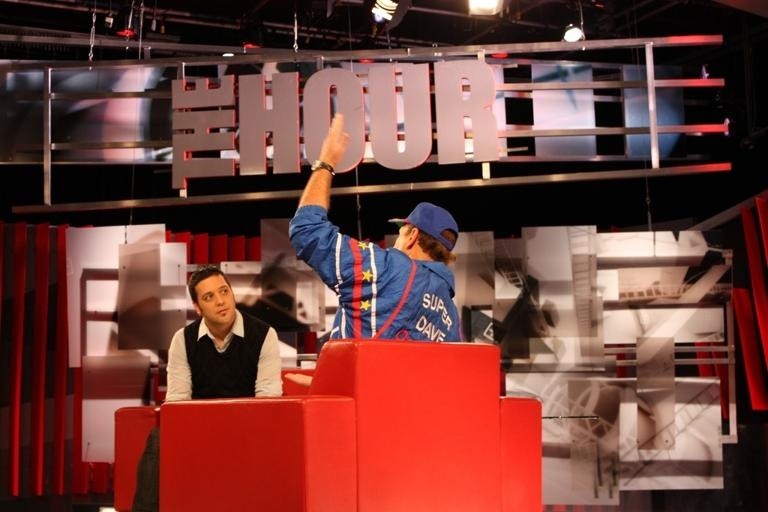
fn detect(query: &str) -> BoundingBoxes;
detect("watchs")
[307,156,339,178]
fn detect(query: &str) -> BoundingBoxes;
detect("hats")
[388,201,459,250]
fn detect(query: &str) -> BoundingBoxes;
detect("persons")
[166,266,286,402]
[288,113,465,342]
